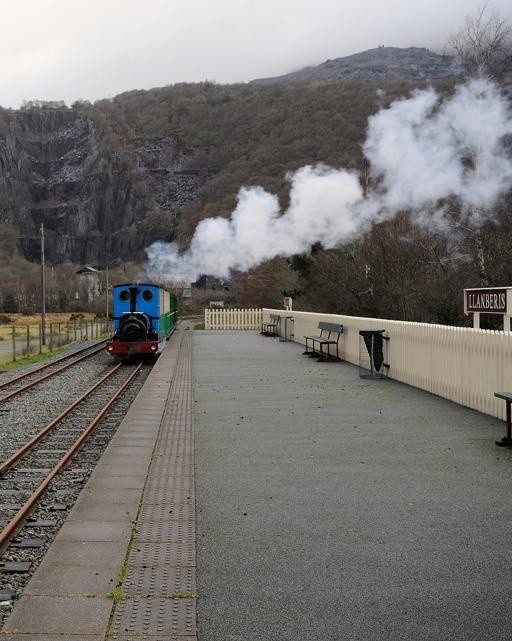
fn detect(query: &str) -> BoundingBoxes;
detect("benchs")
[260,315,279,336]
[494,391,512,446]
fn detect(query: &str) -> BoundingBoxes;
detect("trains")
[105,280,179,356]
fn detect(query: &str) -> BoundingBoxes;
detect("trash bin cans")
[358,329,385,379]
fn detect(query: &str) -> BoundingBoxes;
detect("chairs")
[303,321,343,362]
